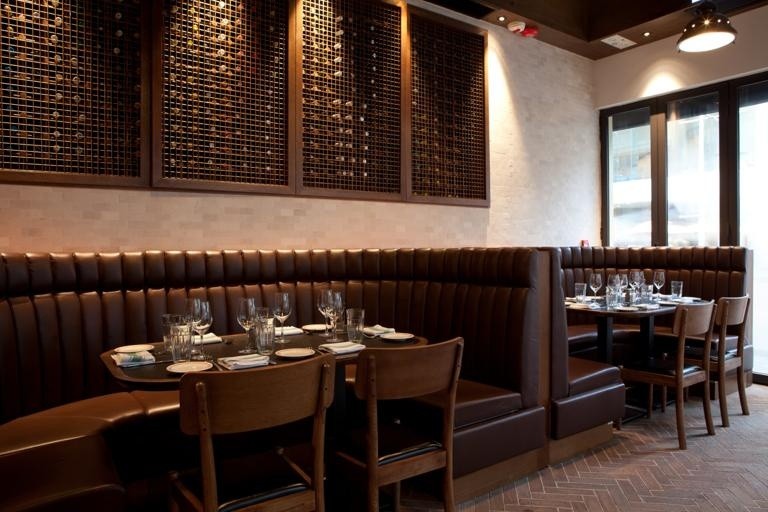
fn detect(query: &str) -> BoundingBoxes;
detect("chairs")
[334,335,465,511]
[660,296,749,427]
[614,298,715,449]
[164,350,336,512]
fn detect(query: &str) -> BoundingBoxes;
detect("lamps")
[675,0,737,54]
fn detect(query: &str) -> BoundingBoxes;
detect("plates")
[113,344,154,352]
[303,324,329,331]
[379,332,414,341]
[276,348,315,357]
[166,361,213,372]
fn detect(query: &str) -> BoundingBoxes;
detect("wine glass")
[272,291,294,344]
[236,295,258,353]
[326,291,345,343]
[318,288,335,338]
[185,297,213,360]
[589,271,666,302]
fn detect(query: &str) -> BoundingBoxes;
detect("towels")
[357,324,395,338]
[317,340,366,354]
[272,325,304,337]
[110,350,156,368]
[217,353,270,370]
[191,331,222,344]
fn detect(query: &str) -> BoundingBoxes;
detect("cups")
[346,308,365,345]
[246,306,270,349]
[159,314,184,352]
[169,324,191,364]
[254,316,277,357]
[566,281,684,312]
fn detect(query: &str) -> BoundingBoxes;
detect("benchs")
[536,245,753,466]
[0,246,549,510]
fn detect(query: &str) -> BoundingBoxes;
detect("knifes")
[121,359,173,368]
[210,359,223,372]
